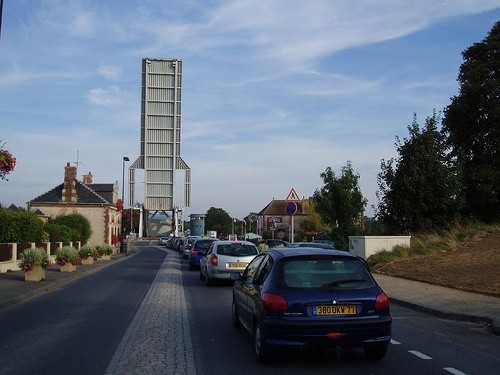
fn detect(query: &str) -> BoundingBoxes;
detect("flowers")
[55,246,81,265]
[96,241,113,257]
[17,247,50,272]
[79,243,97,259]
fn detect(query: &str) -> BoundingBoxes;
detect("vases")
[81,256,93,265]
[60,263,76,272]
[100,253,110,260]
[24,266,46,282]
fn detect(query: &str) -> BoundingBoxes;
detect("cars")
[196,240,260,286]
[229,247,393,364]
[187,238,221,270]
[159,235,203,259]
